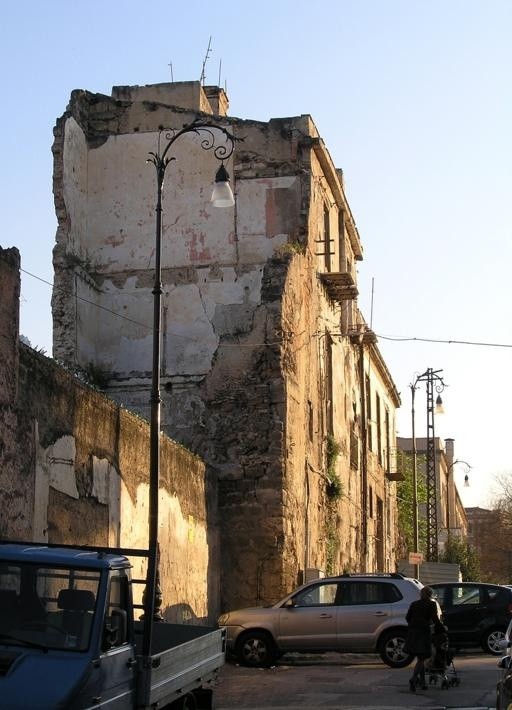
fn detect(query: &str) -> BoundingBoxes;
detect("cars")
[422,582,511,657]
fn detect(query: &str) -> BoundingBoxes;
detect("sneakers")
[409,679,417,691]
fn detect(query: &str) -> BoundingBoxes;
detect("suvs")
[220,568,434,667]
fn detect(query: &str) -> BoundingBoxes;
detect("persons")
[405,586,447,692]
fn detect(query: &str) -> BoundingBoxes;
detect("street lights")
[444,457,474,572]
[142,122,245,619]
[408,370,448,578]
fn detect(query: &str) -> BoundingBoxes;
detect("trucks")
[1,542,227,710]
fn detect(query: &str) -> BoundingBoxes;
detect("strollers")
[407,628,461,694]
